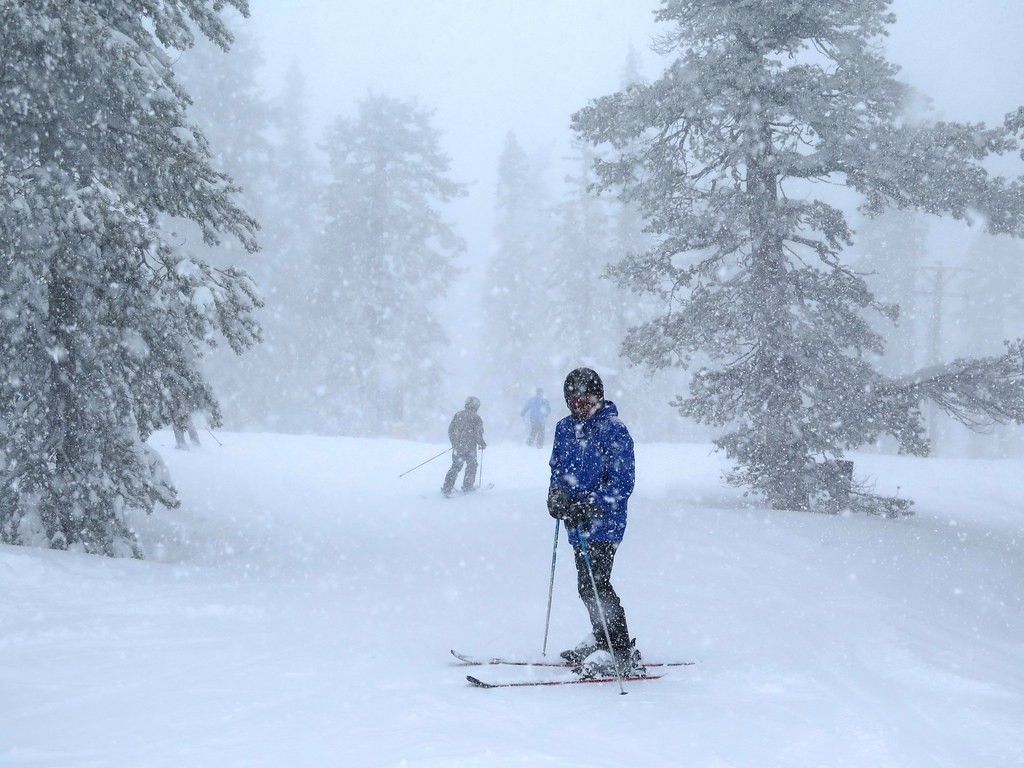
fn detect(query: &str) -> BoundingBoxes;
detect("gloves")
[548,486,572,521]
[564,497,602,526]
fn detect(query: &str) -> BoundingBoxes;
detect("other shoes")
[462,486,476,492]
[561,635,608,665]
[443,487,457,494]
[571,637,647,679]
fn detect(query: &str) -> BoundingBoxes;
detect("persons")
[442,396,487,496]
[518,387,552,449]
[549,367,636,676]
[170,394,201,450]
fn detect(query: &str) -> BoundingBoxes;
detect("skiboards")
[451,648,695,690]
[440,482,495,499]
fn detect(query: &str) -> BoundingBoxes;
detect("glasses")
[567,401,595,411]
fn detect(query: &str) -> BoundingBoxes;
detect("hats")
[464,397,481,410]
[564,369,606,398]
